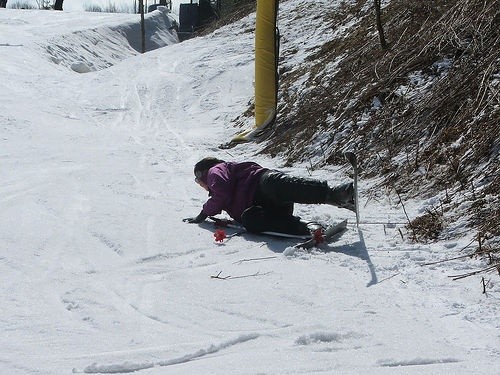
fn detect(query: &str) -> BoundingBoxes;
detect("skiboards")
[293,152,359,252]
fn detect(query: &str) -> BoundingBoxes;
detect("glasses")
[194,168,203,185]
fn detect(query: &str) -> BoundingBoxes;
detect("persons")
[181,157,356,236]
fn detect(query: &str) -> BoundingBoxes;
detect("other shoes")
[297,218,326,237]
[332,179,354,204]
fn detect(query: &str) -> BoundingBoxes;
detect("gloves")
[183,210,207,223]
